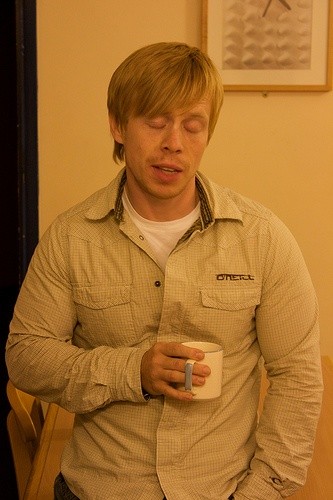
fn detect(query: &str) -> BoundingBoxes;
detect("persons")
[5,47,323,500]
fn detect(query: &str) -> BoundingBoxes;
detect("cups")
[174,340,223,402]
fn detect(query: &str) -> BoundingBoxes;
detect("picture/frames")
[200,0,333,92]
